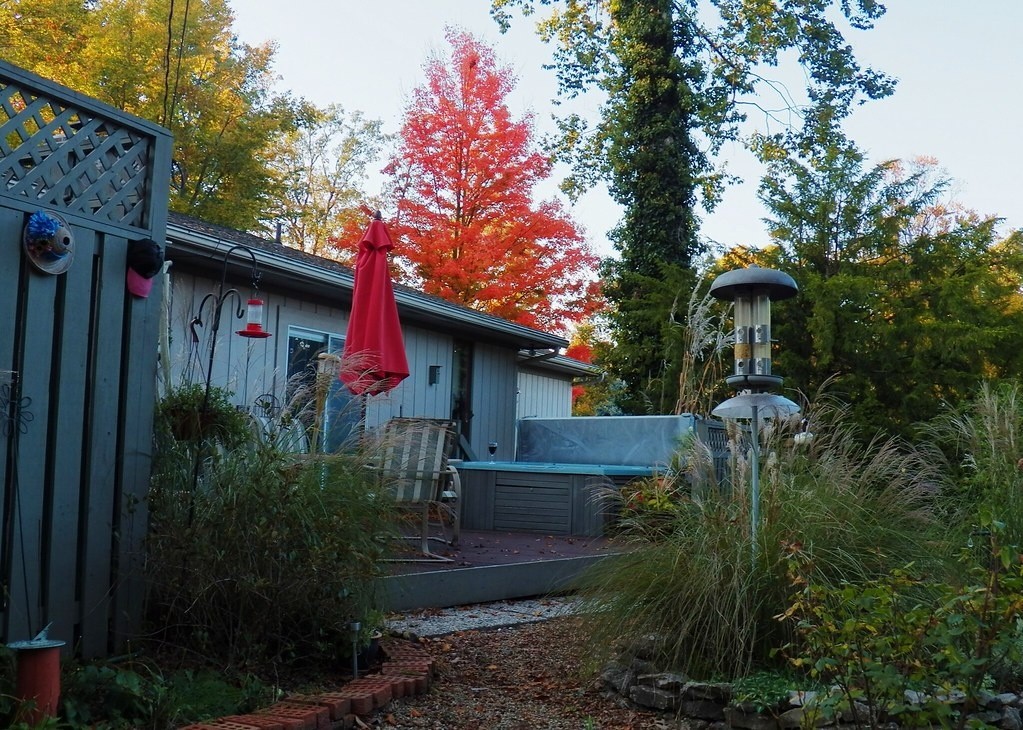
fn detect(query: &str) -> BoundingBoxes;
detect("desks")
[293,454,463,554]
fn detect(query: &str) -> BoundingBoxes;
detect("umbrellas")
[338,210,411,474]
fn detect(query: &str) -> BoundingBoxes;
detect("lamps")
[346,621,363,644]
[707,261,798,388]
[235,287,273,339]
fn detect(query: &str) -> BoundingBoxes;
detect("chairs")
[240,404,310,453]
[370,416,457,564]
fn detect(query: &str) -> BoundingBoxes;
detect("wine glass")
[487,441,498,464]
